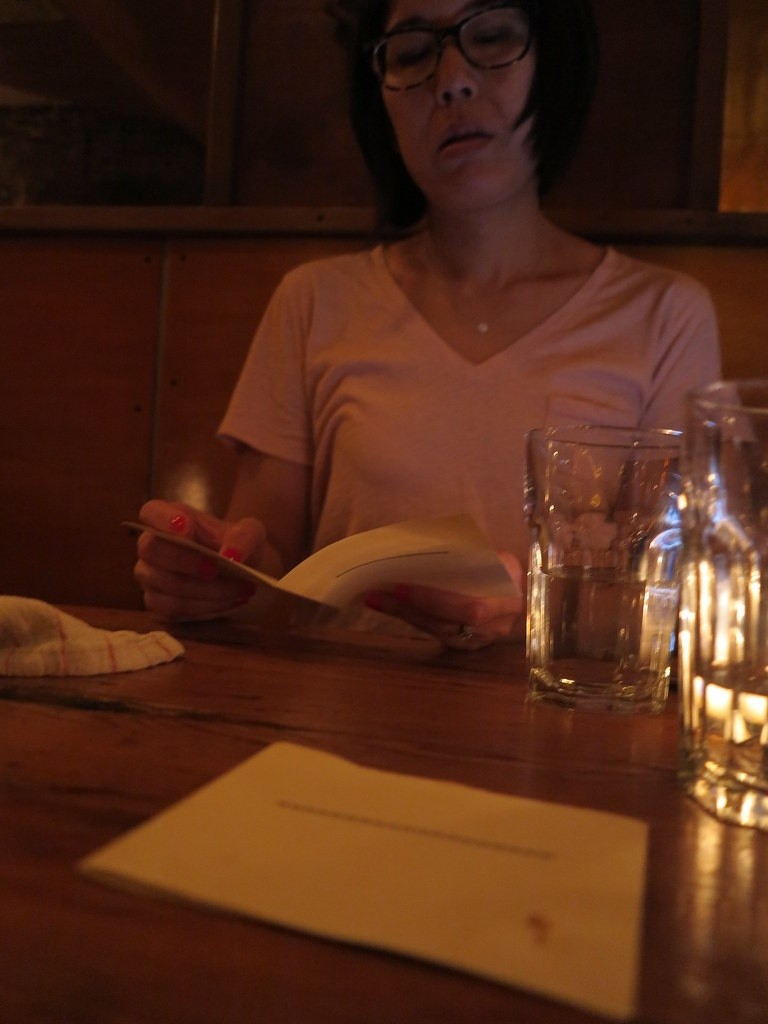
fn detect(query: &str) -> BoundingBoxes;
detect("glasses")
[373,5,541,92]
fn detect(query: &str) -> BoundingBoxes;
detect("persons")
[129,2,726,682]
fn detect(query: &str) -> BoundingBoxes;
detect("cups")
[676,375,768,834]
[523,423,692,715]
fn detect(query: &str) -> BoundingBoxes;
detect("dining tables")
[0,581,768,1024]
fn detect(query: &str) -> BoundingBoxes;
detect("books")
[75,740,654,1024]
[124,507,522,627]
